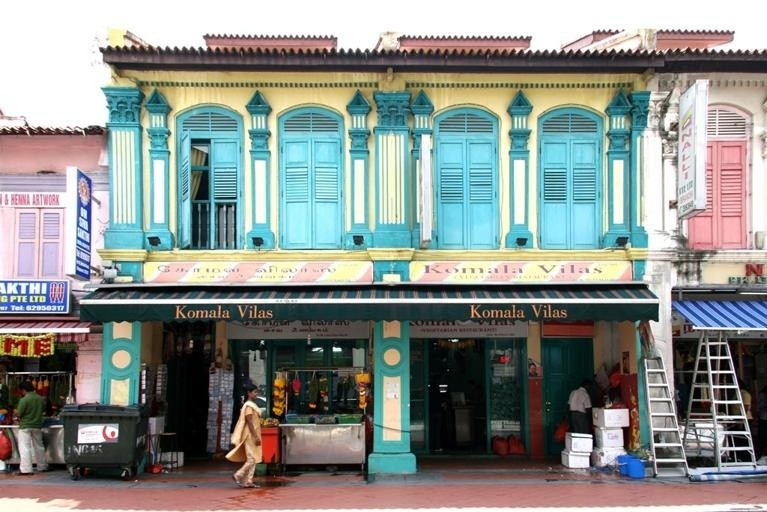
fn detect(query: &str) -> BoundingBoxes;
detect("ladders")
[644,355,691,478]
[683,331,758,472]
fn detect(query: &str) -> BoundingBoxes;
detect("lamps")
[616,236,629,247]
[147,236,161,251]
[514,237,529,248]
[251,236,263,251]
[352,235,364,250]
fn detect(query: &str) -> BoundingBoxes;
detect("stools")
[154,432,179,472]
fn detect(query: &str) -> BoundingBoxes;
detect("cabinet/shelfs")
[0,424,69,472]
[278,421,367,480]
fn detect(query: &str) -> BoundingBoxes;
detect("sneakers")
[231,473,261,489]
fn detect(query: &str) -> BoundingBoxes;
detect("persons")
[728,377,758,461]
[565,375,594,431]
[222,381,264,487]
[526,363,538,376]
[11,380,49,476]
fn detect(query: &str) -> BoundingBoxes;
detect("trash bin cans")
[60,404,139,481]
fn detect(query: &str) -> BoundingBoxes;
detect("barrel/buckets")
[627,459,649,478]
[617,455,636,475]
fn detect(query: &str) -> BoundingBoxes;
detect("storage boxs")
[560,407,630,470]
[146,416,184,469]
